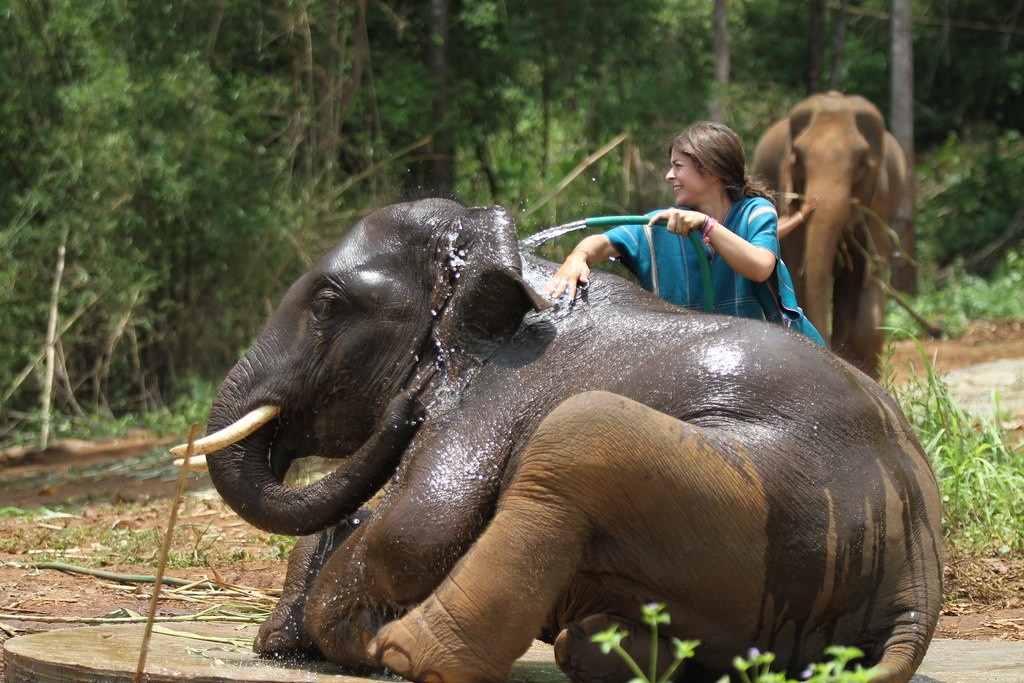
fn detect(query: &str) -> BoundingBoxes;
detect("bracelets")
[797,212,803,220]
[699,215,717,243]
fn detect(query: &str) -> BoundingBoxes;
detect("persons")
[547,122,826,347]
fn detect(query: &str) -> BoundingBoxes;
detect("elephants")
[172,199,942,683]
[752,89,905,382]
